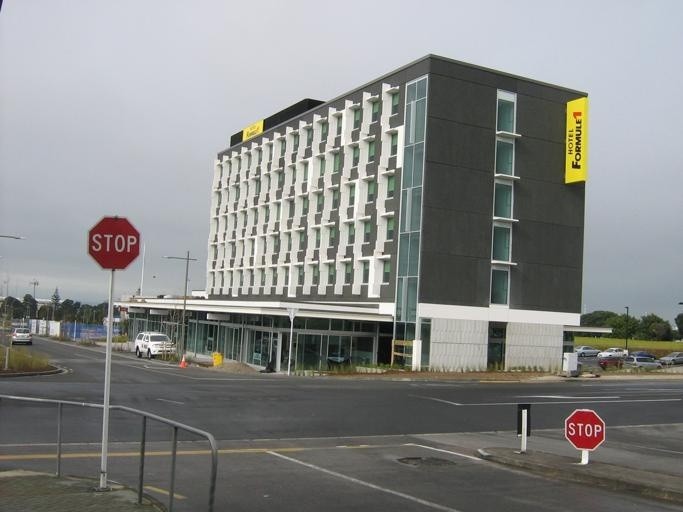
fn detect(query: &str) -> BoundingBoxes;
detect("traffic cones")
[178,353,187,369]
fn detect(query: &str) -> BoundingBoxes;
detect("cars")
[623,350,683,370]
[6,327,33,346]
[596,347,629,359]
[575,345,601,358]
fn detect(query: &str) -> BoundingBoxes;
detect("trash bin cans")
[213,353,223,366]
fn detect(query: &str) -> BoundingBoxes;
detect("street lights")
[161,249,198,363]
[623,306,629,349]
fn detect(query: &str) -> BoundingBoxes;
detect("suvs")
[133,331,177,361]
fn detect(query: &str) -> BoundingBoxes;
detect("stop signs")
[563,408,606,464]
[85,217,141,492]
